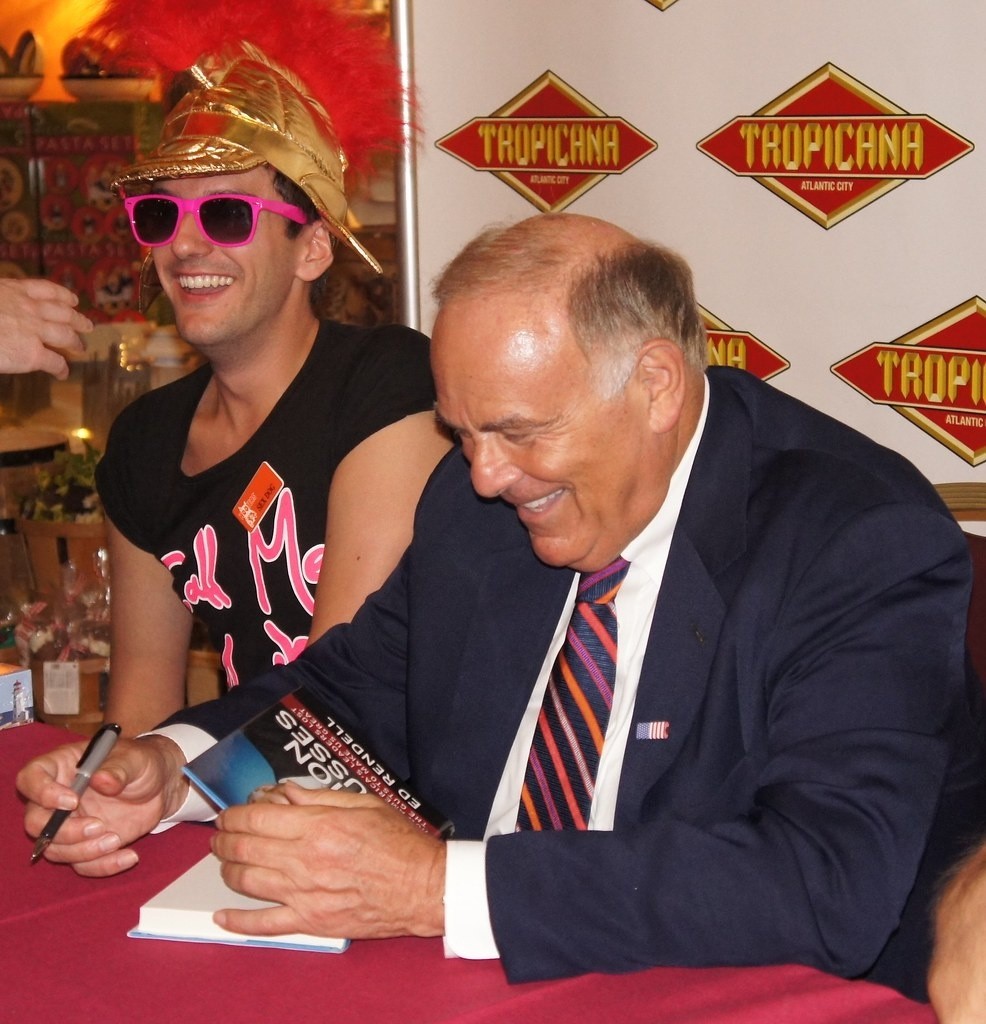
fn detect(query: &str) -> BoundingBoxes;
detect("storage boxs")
[0,99,150,728]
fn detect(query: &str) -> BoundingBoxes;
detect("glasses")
[122,194,308,248]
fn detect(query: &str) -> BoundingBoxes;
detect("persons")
[1,260,95,385]
[929,843,986,1024]
[14,214,986,1005]
[100,57,460,747]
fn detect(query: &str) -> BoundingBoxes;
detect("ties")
[517,555,632,836]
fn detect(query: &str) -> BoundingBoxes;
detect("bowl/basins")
[57,75,155,103]
[1,74,43,102]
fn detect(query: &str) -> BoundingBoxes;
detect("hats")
[108,40,382,276]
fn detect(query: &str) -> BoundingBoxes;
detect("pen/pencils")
[28,722,123,863]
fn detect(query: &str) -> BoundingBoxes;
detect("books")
[125,683,456,954]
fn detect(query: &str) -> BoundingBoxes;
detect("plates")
[0,29,44,75]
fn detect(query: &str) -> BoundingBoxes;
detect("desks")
[0,727,939,1024]
